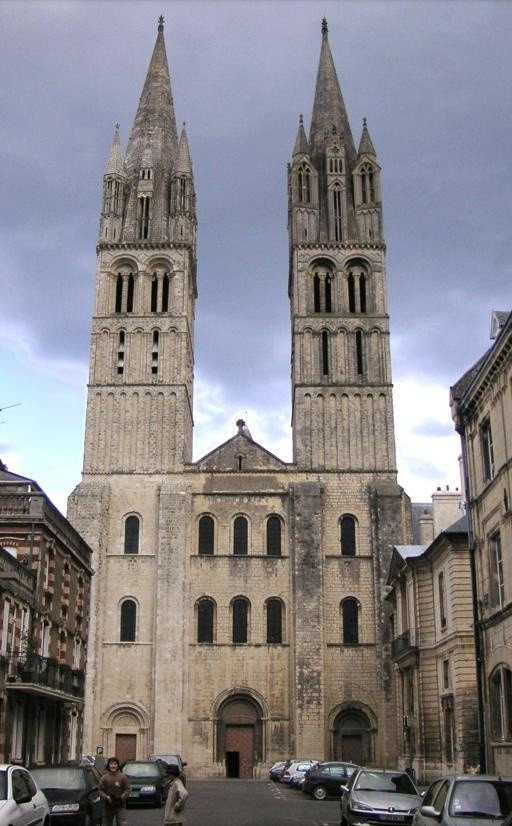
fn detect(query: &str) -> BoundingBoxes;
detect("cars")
[339,766,427,826]
[268,758,359,800]
[148,752,187,788]
[412,773,512,825]
[118,759,170,809]
[0,753,109,826]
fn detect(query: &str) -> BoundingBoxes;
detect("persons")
[162,764,189,826]
[96,757,131,826]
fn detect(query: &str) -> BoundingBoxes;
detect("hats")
[165,764,181,773]
